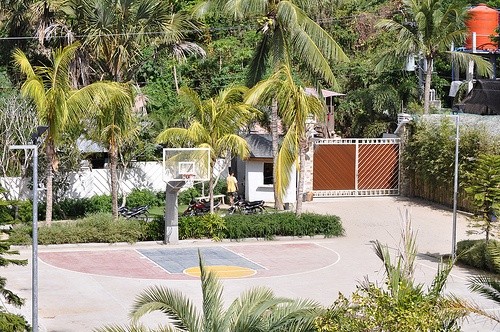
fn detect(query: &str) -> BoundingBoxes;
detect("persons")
[225,170,239,207]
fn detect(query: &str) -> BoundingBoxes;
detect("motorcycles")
[227,197,265,215]
[183,193,221,217]
[117,204,149,224]
